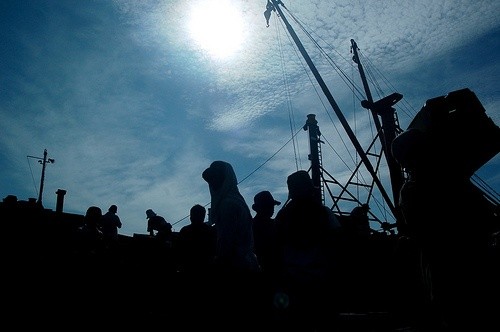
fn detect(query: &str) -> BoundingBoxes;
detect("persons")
[173,161,339,332]
[85,206,120,237]
[147,210,170,240]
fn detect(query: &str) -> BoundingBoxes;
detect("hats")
[286,170,320,199]
[252,190,281,211]
[145,208,157,219]
[108,204,118,214]
[188,204,206,223]
